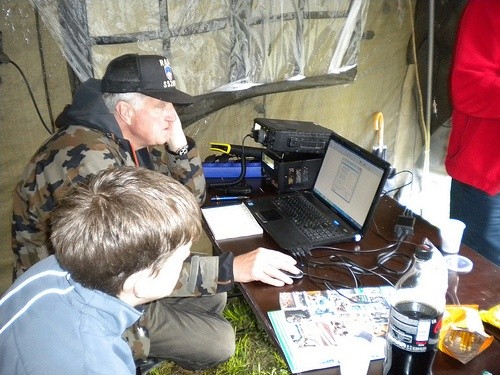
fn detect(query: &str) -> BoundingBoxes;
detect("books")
[201,204,264,242]
[268,286,403,374]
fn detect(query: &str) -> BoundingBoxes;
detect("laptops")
[245,131,392,253]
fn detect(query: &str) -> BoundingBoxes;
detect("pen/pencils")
[210,196,250,200]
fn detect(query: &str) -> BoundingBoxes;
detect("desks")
[200,186,500,375]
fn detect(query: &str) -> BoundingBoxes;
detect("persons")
[0,54,299,370]
[0,169,200,375]
[445,0,500,265]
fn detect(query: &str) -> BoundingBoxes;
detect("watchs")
[175,144,189,156]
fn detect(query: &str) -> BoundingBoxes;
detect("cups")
[440,219,466,254]
[339,336,373,375]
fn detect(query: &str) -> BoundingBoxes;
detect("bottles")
[382,243,446,375]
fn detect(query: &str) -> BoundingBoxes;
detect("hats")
[102,53,194,105]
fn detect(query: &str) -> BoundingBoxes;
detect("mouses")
[257,268,303,282]
